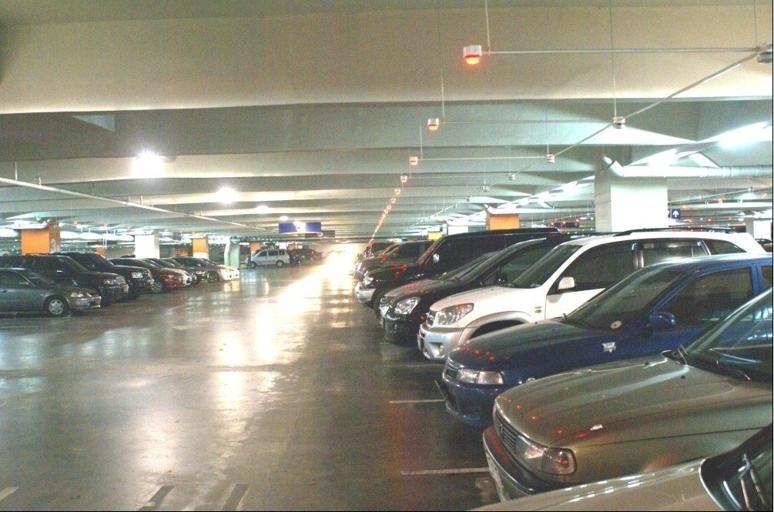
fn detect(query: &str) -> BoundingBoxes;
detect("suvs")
[429,250,773,434]
[350,226,626,345]
[415,223,773,365]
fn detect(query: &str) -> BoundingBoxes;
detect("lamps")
[427,117,440,131]
[755,46,772,65]
[408,155,419,167]
[508,174,515,182]
[481,186,490,193]
[461,44,484,67]
[394,188,401,195]
[400,175,408,182]
[612,116,627,129]
[366,196,473,253]
[545,153,555,164]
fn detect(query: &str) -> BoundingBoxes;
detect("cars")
[463,421,773,512]
[0,251,240,317]
[481,283,773,504]
[245,247,323,268]
